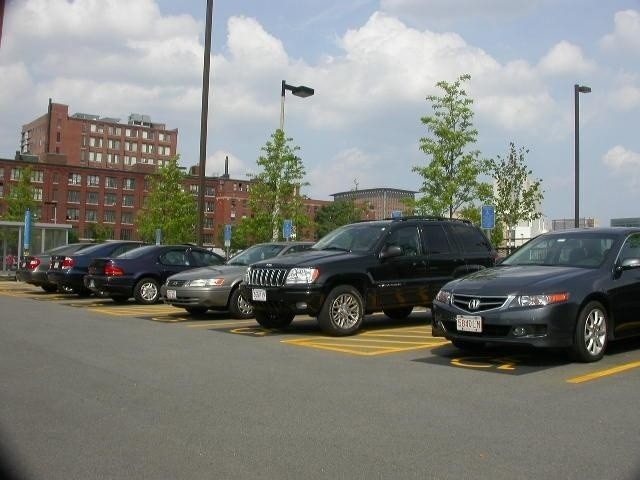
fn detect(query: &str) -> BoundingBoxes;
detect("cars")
[47,241,169,297]
[83,243,230,305]
[16,242,104,294]
[165,242,324,320]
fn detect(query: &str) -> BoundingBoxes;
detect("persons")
[6,247,30,272]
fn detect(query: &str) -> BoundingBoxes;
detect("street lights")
[272,80,315,242]
[575,84,590,227]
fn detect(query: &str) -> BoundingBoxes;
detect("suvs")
[238,213,499,337]
[431,226,640,363]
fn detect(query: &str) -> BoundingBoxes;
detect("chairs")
[543,249,560,266]
